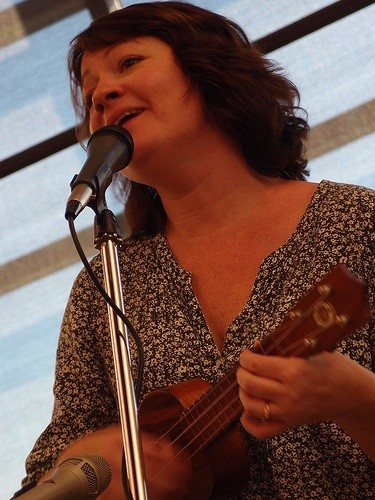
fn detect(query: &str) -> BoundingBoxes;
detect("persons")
[16,2,375,500]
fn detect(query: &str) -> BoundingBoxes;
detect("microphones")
[65,124,134,220]
[11,454,113,500]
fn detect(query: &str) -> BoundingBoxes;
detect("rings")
[263,402,270,421]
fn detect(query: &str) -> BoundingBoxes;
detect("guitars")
[37,263,372,500]
